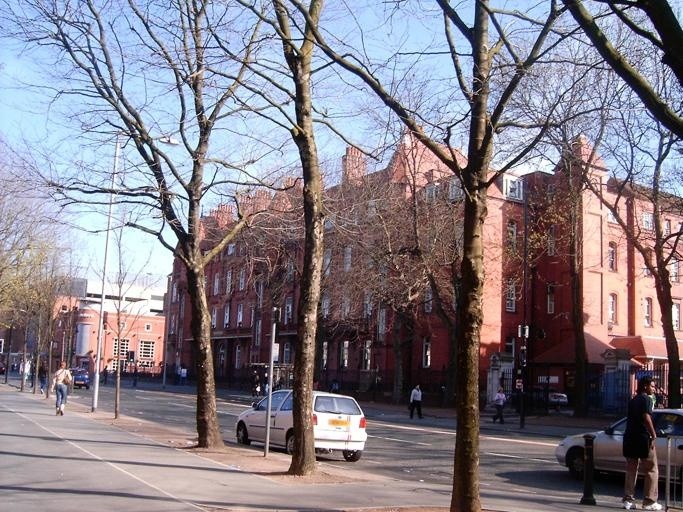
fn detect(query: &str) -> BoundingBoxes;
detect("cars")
[235,388,367,463]
[68,367,91,390]
[554,408,682,489]
[509,385,568,405]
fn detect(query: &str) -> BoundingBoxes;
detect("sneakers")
[642,502,663,510]
[624,500,637,509]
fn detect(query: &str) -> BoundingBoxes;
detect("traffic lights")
[126,351,135,363]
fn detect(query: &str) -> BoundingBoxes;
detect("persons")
[408,383,424,419]
[102,364,107,385]
[12,356,47,395]
[248,372,340,397]
[492,386,506,425]
[621,374,665,510]
[50,361,72,415]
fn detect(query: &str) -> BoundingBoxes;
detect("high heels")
[56,407,64,416]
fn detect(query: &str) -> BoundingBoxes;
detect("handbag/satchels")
[622,431,650,458]
[63,377,70,385]
